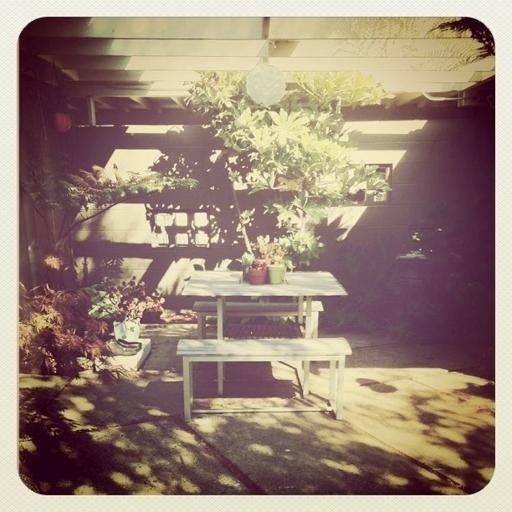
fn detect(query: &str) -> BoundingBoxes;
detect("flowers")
[271,246,288,263]
[93,275,166,323]
[249,258,267,269]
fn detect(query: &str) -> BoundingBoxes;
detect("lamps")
[240,41,288,108]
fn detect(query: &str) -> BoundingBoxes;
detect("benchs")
[176,337,354,423]
[191,300,324,339]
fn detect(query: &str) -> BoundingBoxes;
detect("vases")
[268,265,286,285]
[113,321,141,343]
[246,269,268,285]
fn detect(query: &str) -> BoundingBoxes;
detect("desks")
[181,269,350,399]
